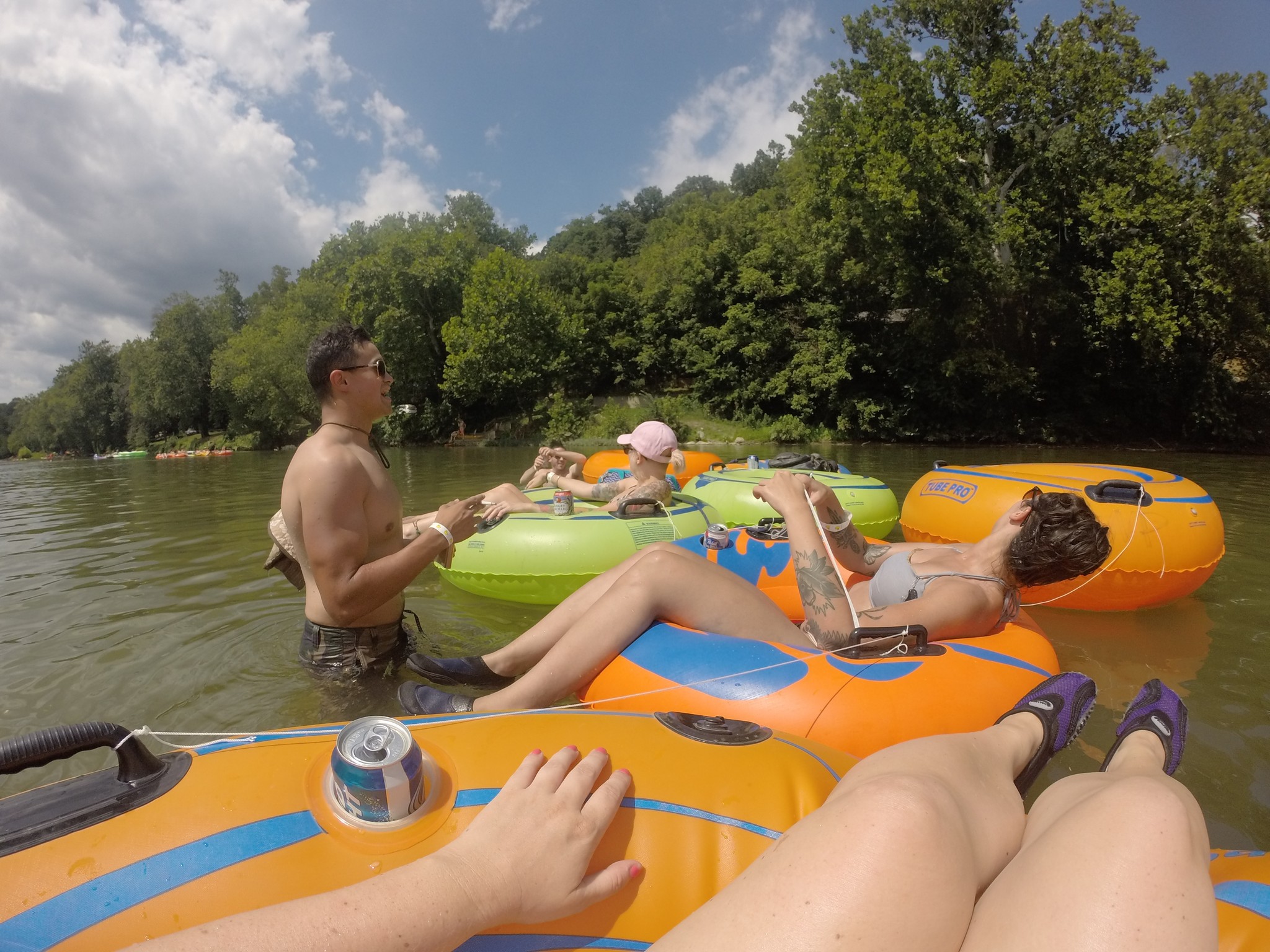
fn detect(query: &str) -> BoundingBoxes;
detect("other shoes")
[1099,679,1188,775]
[993,672,1097,797]
[397,681,477,716]
[403,652,501,683]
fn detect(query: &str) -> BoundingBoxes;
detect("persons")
[458,419,466,438]
[393,471,1112,716]
[449,431,458,443]
[520,439,587,487]
[403,421,685,550]
[280,319,486,680]
[106,670,1219,952]
[157,447,228,458]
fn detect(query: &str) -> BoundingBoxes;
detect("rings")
[526,484,528,487]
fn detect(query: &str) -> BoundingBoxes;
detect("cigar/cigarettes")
[478,501,497,505]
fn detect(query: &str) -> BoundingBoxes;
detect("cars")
[186,428,197,436]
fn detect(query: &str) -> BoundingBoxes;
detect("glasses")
[317,360,385,383]
[1020,486,1044,527]
[623,447,648,461]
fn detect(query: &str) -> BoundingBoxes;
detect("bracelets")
[545,472,550,482]
[533,465,539,472]
[557,475,561,488]
[547,472,556,484]
[429,522,454,546]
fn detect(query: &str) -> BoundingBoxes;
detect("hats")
[617,421,678,464]
